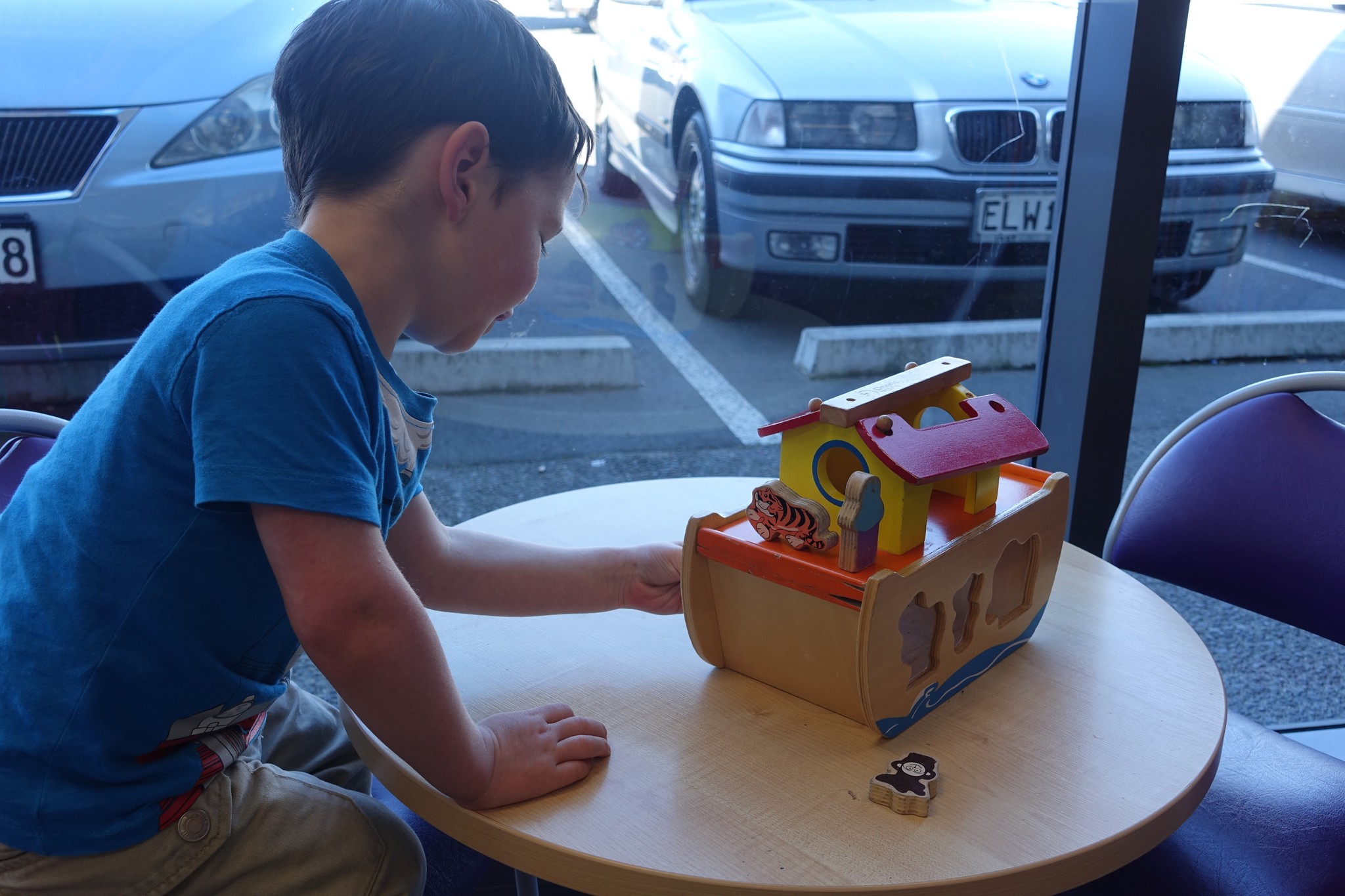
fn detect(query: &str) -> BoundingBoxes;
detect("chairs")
[1102,366,1342,896]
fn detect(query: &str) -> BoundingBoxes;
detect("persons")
[0,0,684,896]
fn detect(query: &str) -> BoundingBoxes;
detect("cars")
[574,0,1273,318]
[0,4,323,366]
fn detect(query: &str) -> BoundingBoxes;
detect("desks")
[335,467,1236,893]
